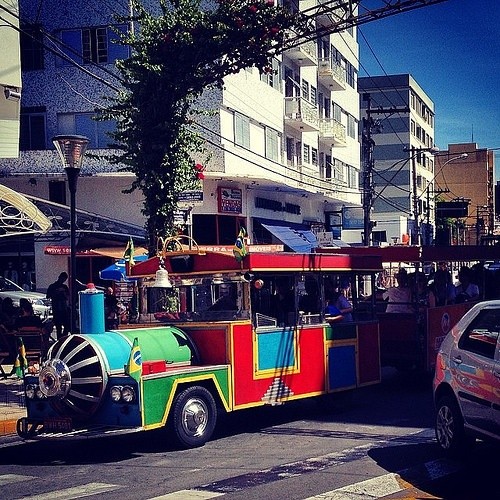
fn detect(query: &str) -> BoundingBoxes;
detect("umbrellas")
[98,256,148,325]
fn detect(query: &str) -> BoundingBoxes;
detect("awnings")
[88,247,150,259]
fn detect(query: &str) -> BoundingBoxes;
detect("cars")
[433,299,500,455]
[0,275,53,319]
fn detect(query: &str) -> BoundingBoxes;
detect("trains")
[15,243,500,447]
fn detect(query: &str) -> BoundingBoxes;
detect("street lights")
[417,153,468,199]
[51,134,92,333]
[369,147,440,212]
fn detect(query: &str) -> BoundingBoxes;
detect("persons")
[46,272,71,339]
[0,297,41,364]
[206,262,479,323]
[3,261,31,291]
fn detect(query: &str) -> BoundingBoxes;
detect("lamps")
[152,259,173,290]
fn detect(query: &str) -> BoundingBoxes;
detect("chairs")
[0,325,48,380]
